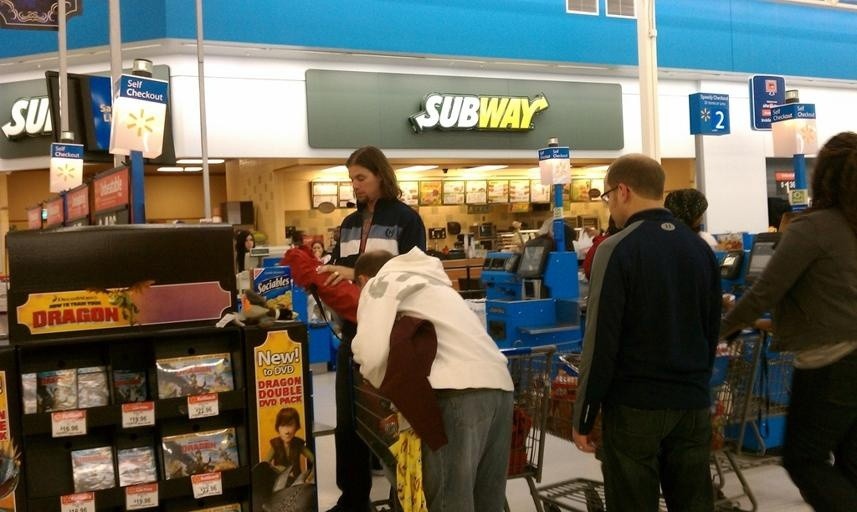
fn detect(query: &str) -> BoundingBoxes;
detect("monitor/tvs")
[45,71,177,168]
[515,238,550,277]
[747,239,780,280]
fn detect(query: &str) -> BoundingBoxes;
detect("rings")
[334,272,338,276]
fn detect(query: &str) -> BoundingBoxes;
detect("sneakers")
[326,499,377,512]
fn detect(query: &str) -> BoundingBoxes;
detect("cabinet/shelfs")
[15,321,251,512]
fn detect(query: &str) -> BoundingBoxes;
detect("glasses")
[601,185,630,203]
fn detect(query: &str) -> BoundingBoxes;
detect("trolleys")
[350,341,561,512]
[530,316,768,512]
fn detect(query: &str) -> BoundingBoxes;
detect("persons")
[315,143,426,512]
[763,194,798,233]
[570,155,721,511]
[664,186,720,250]
[264,409,315,484]
[310,242,333,263]
[584,217,604,281]
[323,222,344,267]
[721,130,857,511]
[352,244,513,511]
[234,229,254,274]
